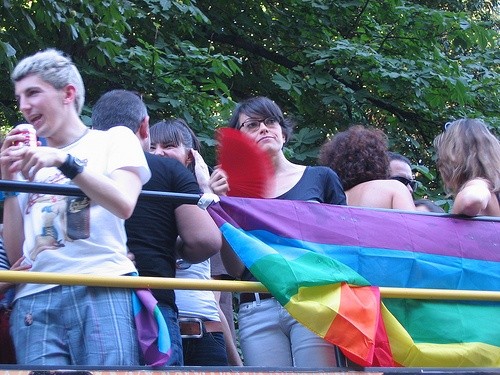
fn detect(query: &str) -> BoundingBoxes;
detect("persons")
[149,117,245,366]
[92,89,222,366]
[0,47,153,367]
[209,97,352,367]
[315,125,419,368]
[385,151,417,199]
[433,119,500,216]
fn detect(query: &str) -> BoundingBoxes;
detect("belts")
[177,317,223,338]
[240,291,273,304]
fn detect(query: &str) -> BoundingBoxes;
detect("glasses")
[238,115,278,131]
[442,122,452,134]
[390,177,417,190]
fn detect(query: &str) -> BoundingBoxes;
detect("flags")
[198,193,499,368]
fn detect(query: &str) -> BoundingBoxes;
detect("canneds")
[13,123,37,147]
